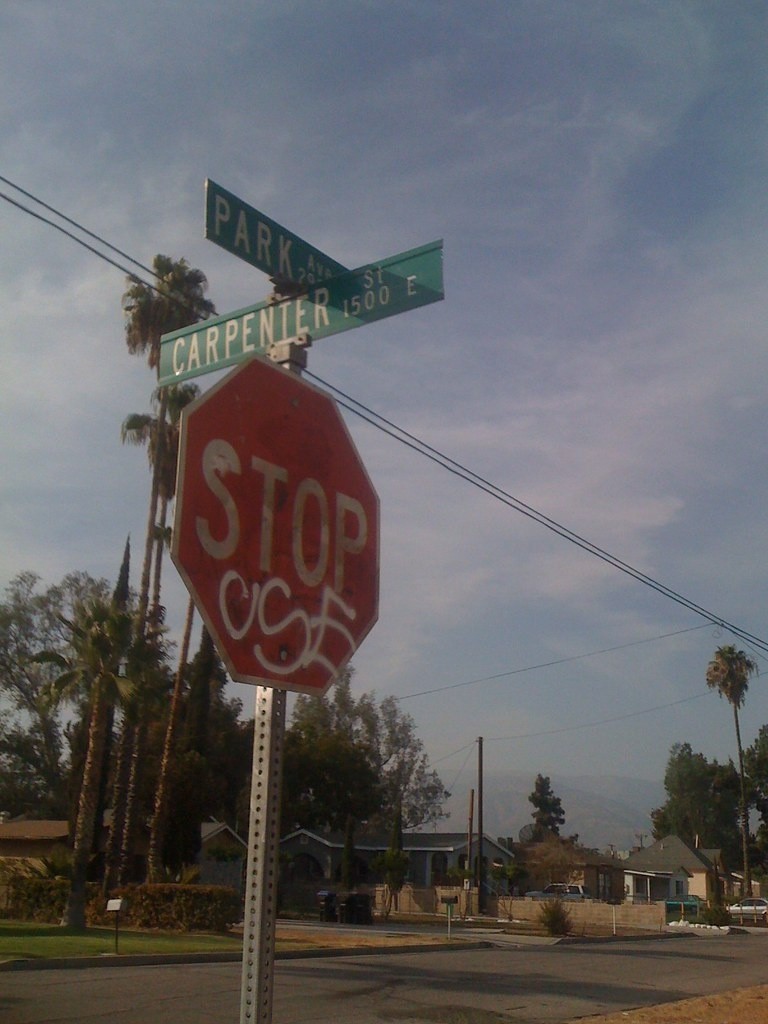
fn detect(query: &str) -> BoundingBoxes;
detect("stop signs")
[166,350,382,699]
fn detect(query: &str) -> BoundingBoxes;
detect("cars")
[723,897,768,921]
[661,893,710,913]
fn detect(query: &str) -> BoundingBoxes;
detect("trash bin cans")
[316,890,337,922]
[344,894,373,925]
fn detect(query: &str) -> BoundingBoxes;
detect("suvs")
[524,883,587,902]
